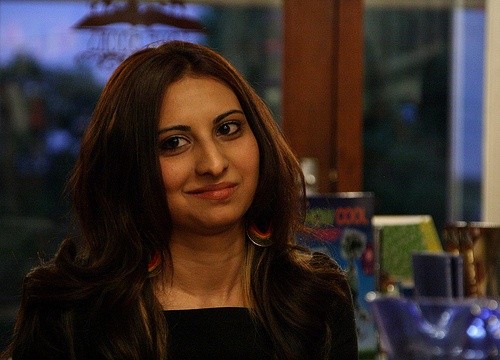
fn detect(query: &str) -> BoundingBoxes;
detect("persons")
[0,36,359,360]
